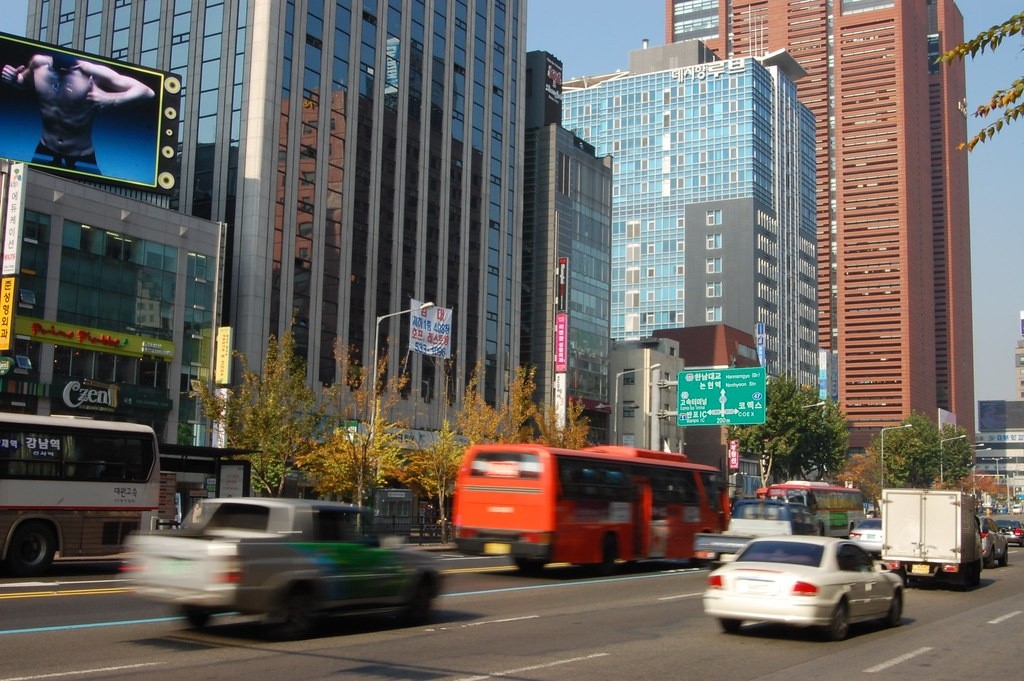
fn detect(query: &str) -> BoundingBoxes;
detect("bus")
[0,413,160,577]
[764,480,865,537]
[452,445,742,578]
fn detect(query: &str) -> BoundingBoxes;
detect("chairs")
[89,461,105,478]
[743,507,755,519]
[767,507,778,519]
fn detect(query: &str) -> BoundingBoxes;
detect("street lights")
[369,301,435,447]
[882,424,913,486]
[941,435,966,483]
[615,363,662,446]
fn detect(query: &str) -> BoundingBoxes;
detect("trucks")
[881,488,983,591]
[692,500,820,571]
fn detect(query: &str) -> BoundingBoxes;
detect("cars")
[994,519,1024,547]
[117,498,448,641]
[977,514,1009,568]
[848,519,881,558]
[702,535,904,641]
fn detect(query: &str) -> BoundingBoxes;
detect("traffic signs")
[676,366,767,427]
